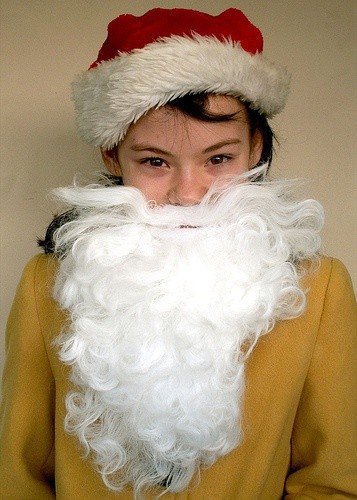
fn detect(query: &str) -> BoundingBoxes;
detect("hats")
[72,7,290,150]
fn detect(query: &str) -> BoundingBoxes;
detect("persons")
[0,6,357,500]
